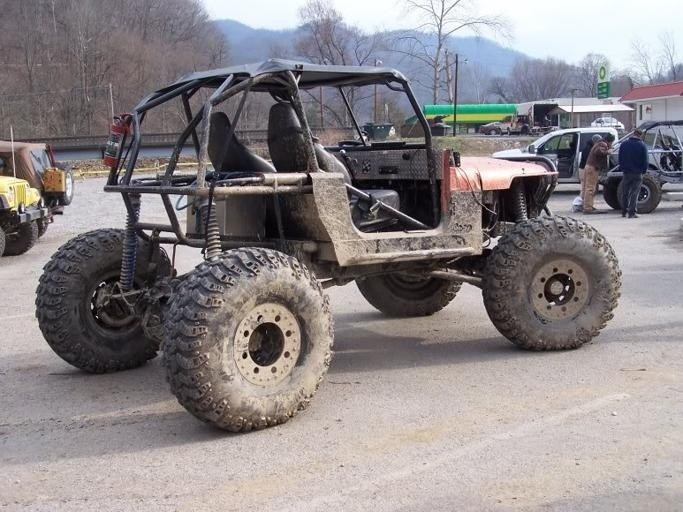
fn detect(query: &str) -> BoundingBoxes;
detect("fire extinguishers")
[102,113,135,168]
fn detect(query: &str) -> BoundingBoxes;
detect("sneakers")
[582,207,600,214]
[628,215,638,218]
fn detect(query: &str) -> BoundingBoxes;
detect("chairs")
[554,142,576,178]
[206,113,293,239]
[266,103,401,232]
[655,137,682,171]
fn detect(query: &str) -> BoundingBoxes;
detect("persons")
[573,133,614,213]
[618,128,648,218]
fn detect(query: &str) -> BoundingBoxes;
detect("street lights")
[371,57,385,125]
[450,52,470,137]
[316,55,330,128]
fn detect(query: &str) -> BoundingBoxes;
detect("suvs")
[33,54,628,434]
[477,112,682,215]
[0,139,75,239]
[0,175,54,259]
